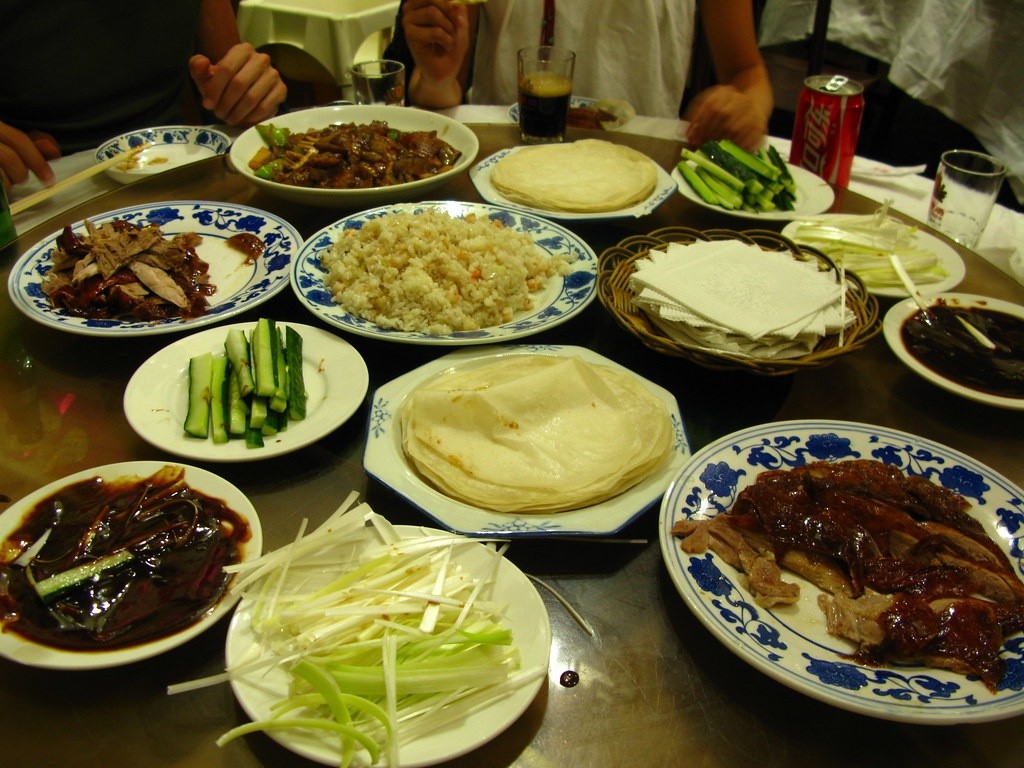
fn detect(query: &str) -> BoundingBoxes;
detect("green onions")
[165,487,652,768]
[792,198,949,319]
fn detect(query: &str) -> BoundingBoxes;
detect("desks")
[236,0,400,103]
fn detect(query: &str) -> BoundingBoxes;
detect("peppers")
[249,123,306,181]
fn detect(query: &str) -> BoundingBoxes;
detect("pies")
[402,355,674,515]
[491,138,659,213]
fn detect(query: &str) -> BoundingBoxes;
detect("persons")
[403,0,774,153]
[0,0,287,194]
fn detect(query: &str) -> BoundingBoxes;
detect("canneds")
[788,75,864,190]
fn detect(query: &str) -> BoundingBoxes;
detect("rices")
[321,208,579,335]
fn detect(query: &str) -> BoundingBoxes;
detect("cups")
[350,60,407,107]
[928,149,1008,256]
[517,45,574,143]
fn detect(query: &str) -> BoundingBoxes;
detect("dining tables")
[0,103,1024,768]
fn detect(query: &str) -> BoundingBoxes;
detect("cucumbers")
[36,551,136,602]
[678,138,797,214]
[184,318,305,447]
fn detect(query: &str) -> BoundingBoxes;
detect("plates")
[659,419,1023,727]
[780,213,964,297]
[223,527,553,767]
[1,461,262,671]
[232,104,479,202]
[670,161,834,220]
[124,321,369,464]
[96,128,232,185]
[508,95,634,133]
[883,294,1024,411]
[7,200,304,336]
[361,346,692,533]
[470,147,679,220]
[286,200,599,347]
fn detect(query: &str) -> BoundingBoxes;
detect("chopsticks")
[12,143,153,214]
